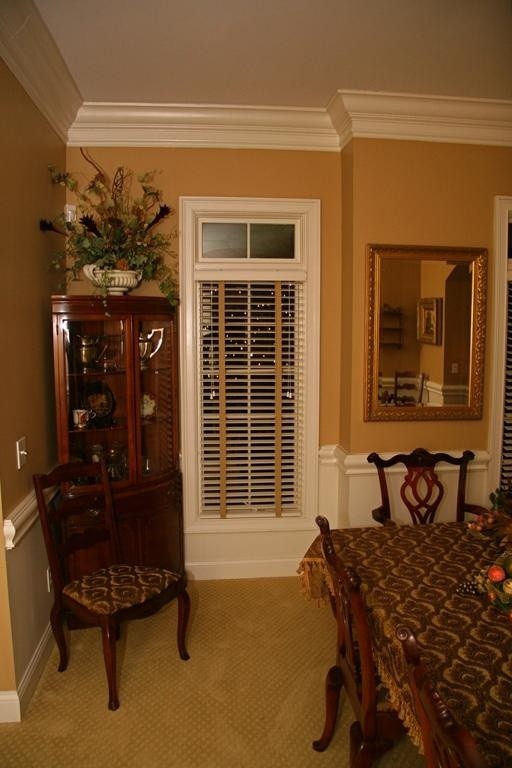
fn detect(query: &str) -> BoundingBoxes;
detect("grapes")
[455,581,478,595]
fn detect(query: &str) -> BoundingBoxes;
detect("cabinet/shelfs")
[380,302,405,350]
[48,293,188,629]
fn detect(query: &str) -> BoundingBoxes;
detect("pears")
[503,578,512,594]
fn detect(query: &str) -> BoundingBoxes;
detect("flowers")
[39,159,185,317]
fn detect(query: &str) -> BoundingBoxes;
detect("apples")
[488,566,506,582]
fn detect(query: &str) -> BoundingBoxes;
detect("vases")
[95,268,141,295]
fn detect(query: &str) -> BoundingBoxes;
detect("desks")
[316,517,512,768]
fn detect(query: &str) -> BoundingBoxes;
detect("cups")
[72,409,97,429]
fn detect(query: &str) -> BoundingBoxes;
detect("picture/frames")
[361,242,490,423]
[414,297,443,346]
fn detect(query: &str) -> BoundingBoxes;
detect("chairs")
[33,458,190,709]
[389,369,426,405]
[311,514,412,767]
[365,446,503,527]
